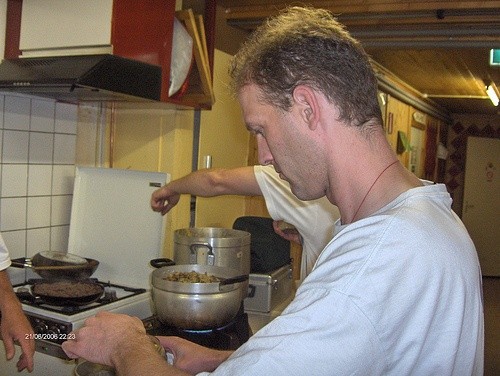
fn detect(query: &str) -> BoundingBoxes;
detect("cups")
[150,335,166,361]
[74,359,117,376]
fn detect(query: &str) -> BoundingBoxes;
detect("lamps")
[485,82,500,107]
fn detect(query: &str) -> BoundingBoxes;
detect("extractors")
[0,54,161,104]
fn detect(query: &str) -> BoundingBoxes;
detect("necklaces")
[351,160,399,223]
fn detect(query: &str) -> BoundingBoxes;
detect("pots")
[9,258,100,281]
[150,257,249,331]
[173,226,251,299]
[10,250,88,266]
[31,279,104,306]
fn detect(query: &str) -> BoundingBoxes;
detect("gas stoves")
[12,279,155,361]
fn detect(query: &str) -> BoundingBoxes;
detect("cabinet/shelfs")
[4,0,218,113]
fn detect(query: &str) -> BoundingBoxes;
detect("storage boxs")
[244,262,293,312]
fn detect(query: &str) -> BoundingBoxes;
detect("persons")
[61,7,485,376]
[0,231,35,373]
[150,164,341,286]
